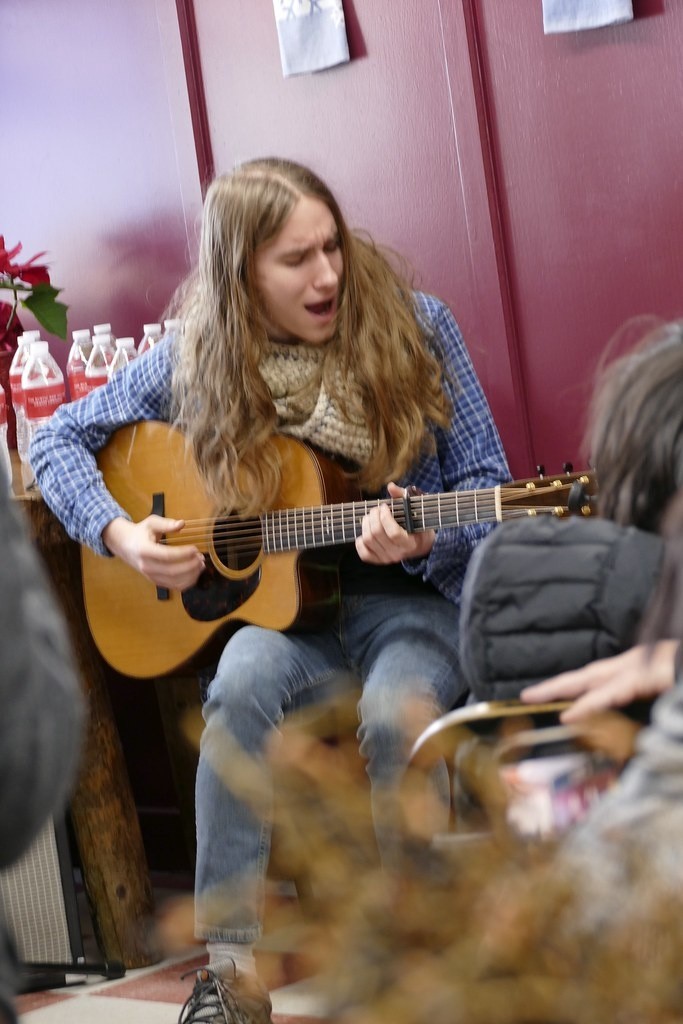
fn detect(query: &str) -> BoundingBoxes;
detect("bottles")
[0,382,13,490]
[21,341,67,444]
[8,330,42,491]
[163,319,178,336]
[137,324,163,356]
[85,323,118,394]
[65,328,94,401]
[109,338,137,383]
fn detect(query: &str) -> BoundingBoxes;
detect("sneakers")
[181,956,276,1024]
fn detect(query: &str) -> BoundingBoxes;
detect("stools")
[178,677,366,778]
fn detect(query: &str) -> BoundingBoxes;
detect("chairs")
[402,701,647,872]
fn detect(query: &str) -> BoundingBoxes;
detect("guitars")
[78,422,612,677]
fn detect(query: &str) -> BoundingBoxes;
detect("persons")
[462,319,683,949]
[29,159,518,1024]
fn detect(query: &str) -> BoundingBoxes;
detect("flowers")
[0,234,69,341]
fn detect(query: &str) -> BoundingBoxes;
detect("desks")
[9,445,168,968]
